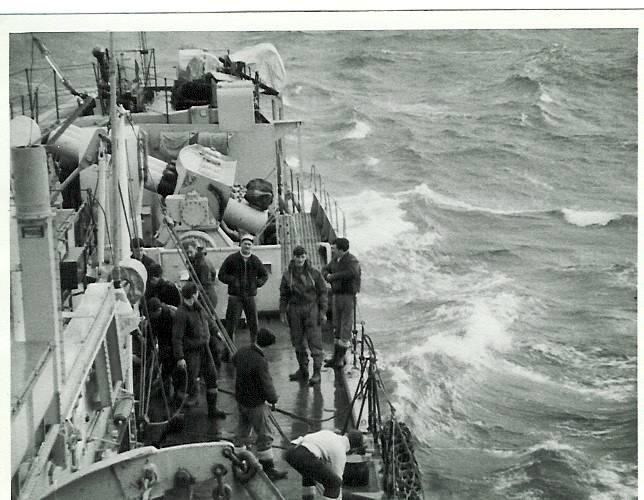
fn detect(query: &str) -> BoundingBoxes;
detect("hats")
[239,233,254,245]
[256,328,276,347]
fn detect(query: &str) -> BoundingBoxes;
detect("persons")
[147,296,187,409]
[181,236,222,370]
[144,262,182,381]
[170,282,227,418]
[129,236,156,278]
[319,238,361,369]
[279,246,328,384]
[217,234,268,357]
[229,328,288,480]
[285,430,364,500]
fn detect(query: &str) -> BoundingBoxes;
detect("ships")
[10,32,429,499]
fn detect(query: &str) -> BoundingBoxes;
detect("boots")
[256,446,287,478]
[206,388,224,418]
[289,338,350,384]
